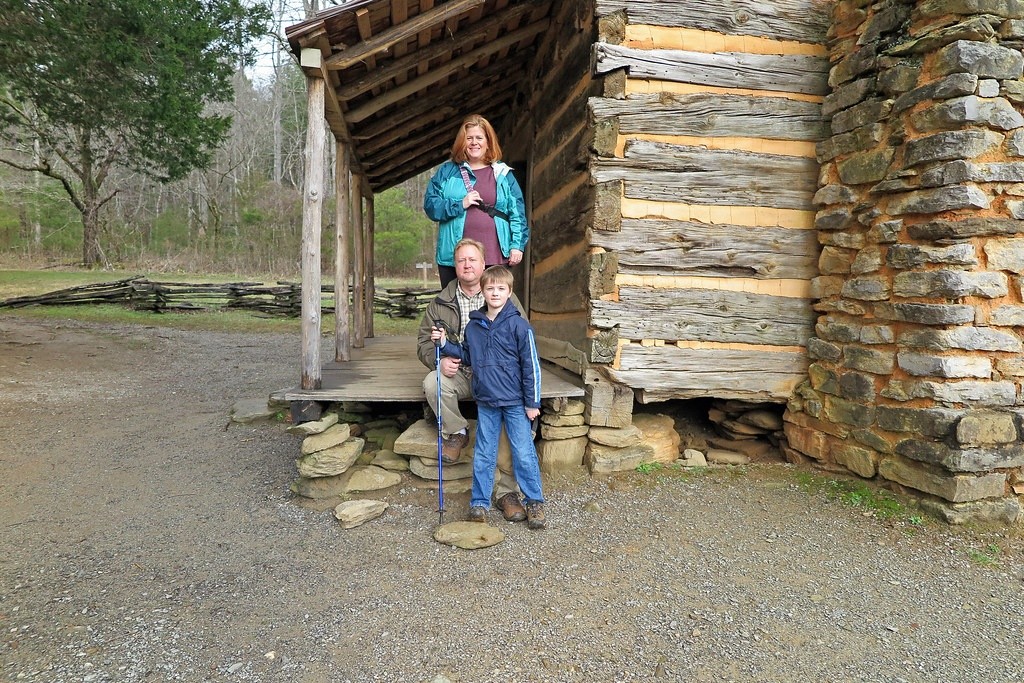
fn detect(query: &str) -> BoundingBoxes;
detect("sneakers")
[470,506,486,522]
[441,427,469,463]
[497,492,527,522]
[525,500,547,529]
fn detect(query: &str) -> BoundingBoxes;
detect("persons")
[430,267,549,529]
[418,239,530,523]
[424,115,528,292]
